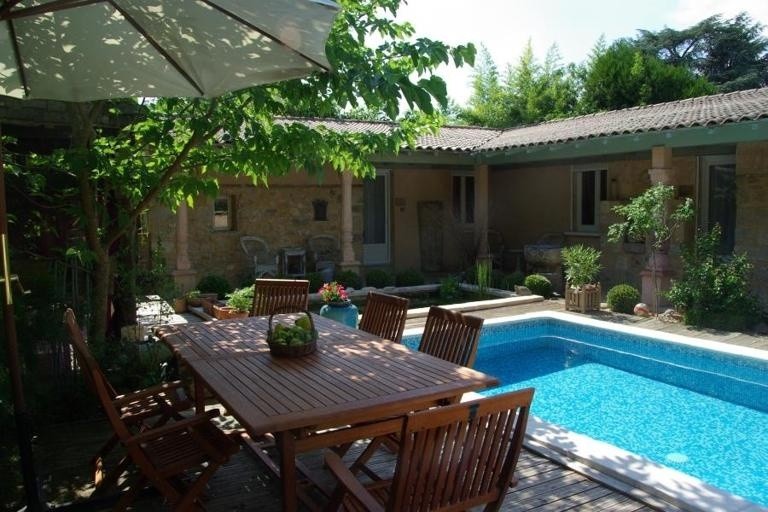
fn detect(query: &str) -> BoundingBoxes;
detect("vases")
[320,298,358,327]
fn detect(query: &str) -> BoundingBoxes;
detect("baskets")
[187,297,204,306]
[267,305,318,360]
[213,305,250,320]
[203,299,225,315]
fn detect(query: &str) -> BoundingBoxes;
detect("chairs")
[322,387,534,511]
[248,278,310,316]
[320,290,410,467]
[347,307,484,475]
[63,308,194,467]
[239,236,281,280]
[64,308,240,511]
[308,236,342,272]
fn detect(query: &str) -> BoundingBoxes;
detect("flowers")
[318,281,351,304]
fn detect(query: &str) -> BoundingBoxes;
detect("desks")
[281,246,306,279]
[150,310,499,512]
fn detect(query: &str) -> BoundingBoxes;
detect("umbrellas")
[0,0,343,512]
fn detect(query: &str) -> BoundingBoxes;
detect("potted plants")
[228,283,255,318]
[560,240,606,313]
